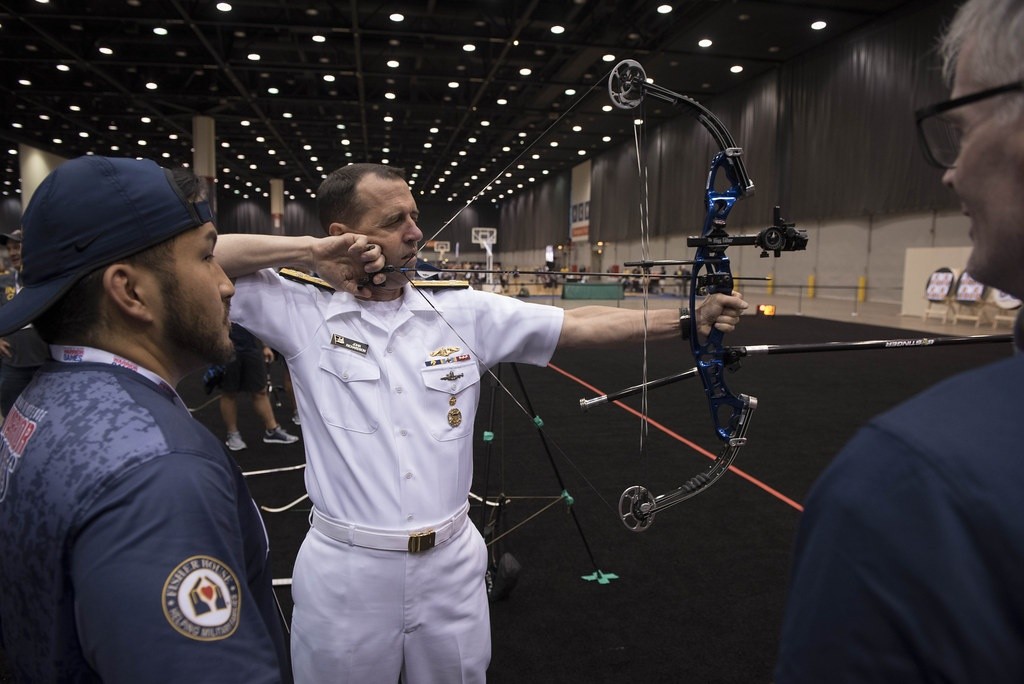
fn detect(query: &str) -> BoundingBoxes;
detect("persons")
[212,163,748,684]
[0,156,291,684]
[777,0,1024,684]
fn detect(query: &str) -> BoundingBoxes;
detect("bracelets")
[679,308,692,340]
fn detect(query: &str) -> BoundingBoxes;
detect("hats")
[0,230,23,246]
[0,156,212,336]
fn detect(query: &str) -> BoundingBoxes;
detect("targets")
[992,287,1023,310]
[925,267,954,302]
[954,268,988,305]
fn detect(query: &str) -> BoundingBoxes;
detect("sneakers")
[226,435,247,450]
[263,426,299,444]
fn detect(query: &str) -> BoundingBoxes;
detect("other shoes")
[292,414,301,425]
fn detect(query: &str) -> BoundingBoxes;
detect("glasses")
[914,80,1024,169]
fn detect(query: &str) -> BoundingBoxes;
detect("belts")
[312,500,470,552]
